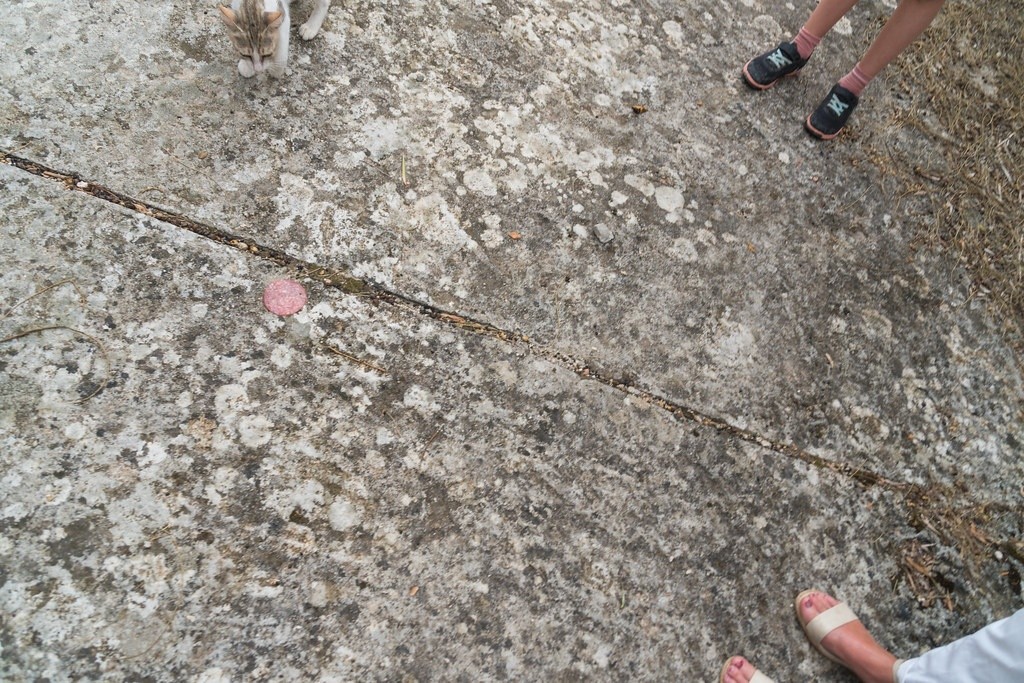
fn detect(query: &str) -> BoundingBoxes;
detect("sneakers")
[807,81,860,140]
[740,39,815,89]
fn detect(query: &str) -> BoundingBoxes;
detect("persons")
[736,0,945,142]
[721,583,1024,682]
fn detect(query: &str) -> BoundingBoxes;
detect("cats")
[218,0,331,79]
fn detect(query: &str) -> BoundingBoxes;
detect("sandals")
[716,655,774,682]
[796,586,909,683]
